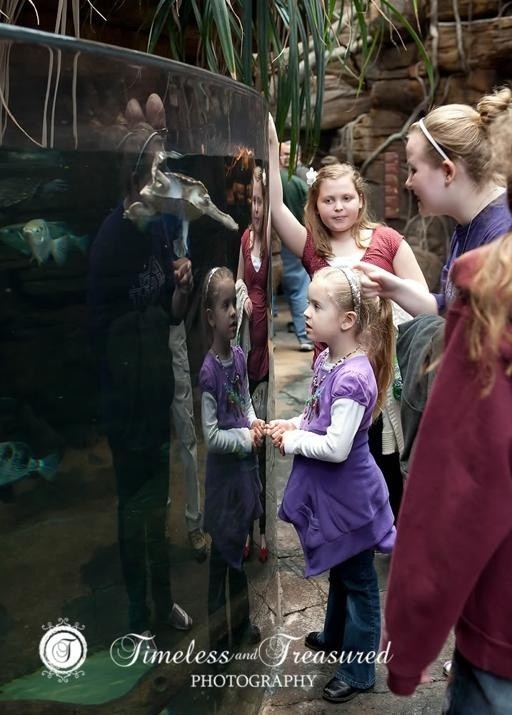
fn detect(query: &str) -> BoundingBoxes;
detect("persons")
[236,166,268,562]
[197,266,262,680]
[268,110,442,531]
[353,84,512,321]
[380,111,512,715]
[271,165,313,351]
[263,263,397,704]
[280,140,314,185]
[84,123,196,669]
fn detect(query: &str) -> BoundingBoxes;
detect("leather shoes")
[304,631,324,652]
[259,546,269,563]
[323,674,374,705]
[244,546,249,559]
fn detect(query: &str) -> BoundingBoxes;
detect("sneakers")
[161,601,194,630]
[189,527,207,564]
[133,629,160,669]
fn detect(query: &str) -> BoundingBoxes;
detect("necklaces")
[310,345,362,394]
[211,345,236,385]
